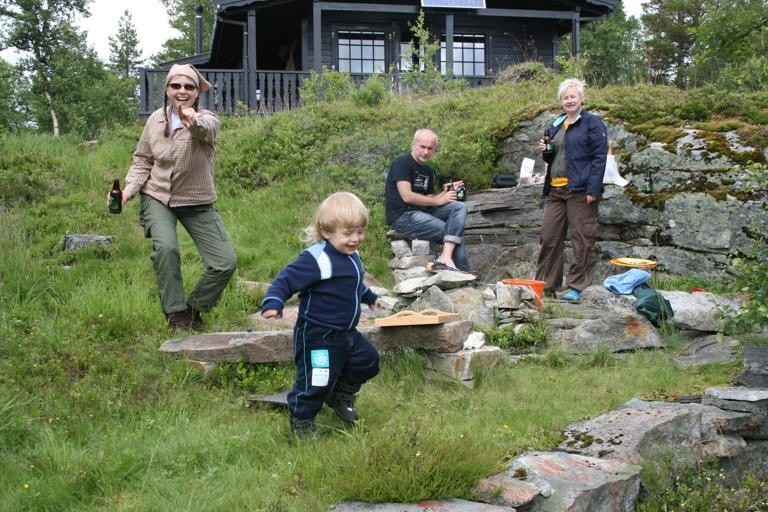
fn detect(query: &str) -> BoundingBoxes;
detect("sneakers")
[166,304,207,334]
[290,415,316,442]
[424,262,479,278]
[561,289,581,300]
[325,397,358,422]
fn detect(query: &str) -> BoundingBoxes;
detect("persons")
[105,62,238,331]
[384,128,468,273]
[261,188,381,438]
[534,77,609,301]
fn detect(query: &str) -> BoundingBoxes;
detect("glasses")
[168,83,197,90]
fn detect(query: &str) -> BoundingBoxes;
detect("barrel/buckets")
[503,279,545,313]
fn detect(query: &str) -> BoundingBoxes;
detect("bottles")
[644,170,653,194]
[542,129,553,162]
[109,179,123,215]
